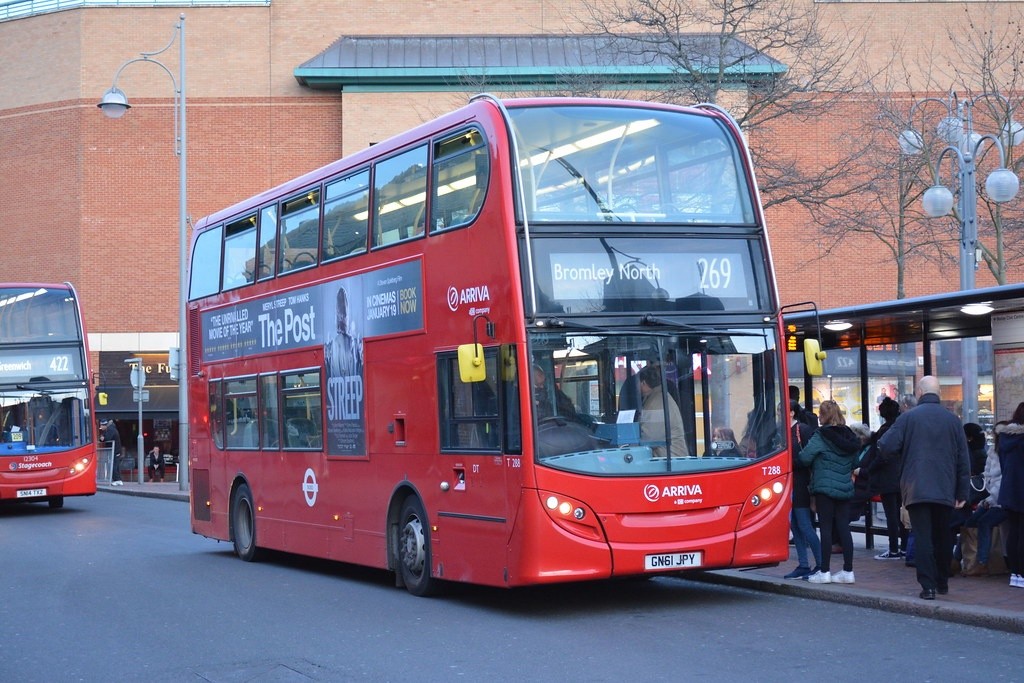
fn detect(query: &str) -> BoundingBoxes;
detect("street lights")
[898,88,1023,427]
[94,12,191,491]
[123,357,145,485]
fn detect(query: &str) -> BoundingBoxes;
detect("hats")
[100,419,108,424]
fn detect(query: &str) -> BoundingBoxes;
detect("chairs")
[225,416,321,449]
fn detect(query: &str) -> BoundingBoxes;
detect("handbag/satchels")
[970,473,990,503]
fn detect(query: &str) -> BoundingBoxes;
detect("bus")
[0,279,98,509]
[180,92,829,599]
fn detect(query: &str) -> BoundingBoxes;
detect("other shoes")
[161,478,165,481]
[149,478,154,482]
[960,565,989,576]
[111,481,123,486]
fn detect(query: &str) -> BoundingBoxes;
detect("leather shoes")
[937,583,946,594]
[920,589,935,599]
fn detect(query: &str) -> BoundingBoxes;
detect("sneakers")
[831,570,855,584]
[874,551,901,560]
[803,566,822,580]
[1009,574,1018,586]
[784,566,811,580]
[807,570,831,584]
[898,549,907,558]
[1016,575,1024,587]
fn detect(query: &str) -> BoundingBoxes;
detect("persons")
[773,386,875,584]
[40,417,58,442]
[148,446,165,481]
[515,360,576,419]
[739,409,781,457]
[703,427,745,456]
[620,352,681,414]
[852,375,971,599]
[636,367,689,456]
[98,418,124,486]
[873,397,1024,588]
